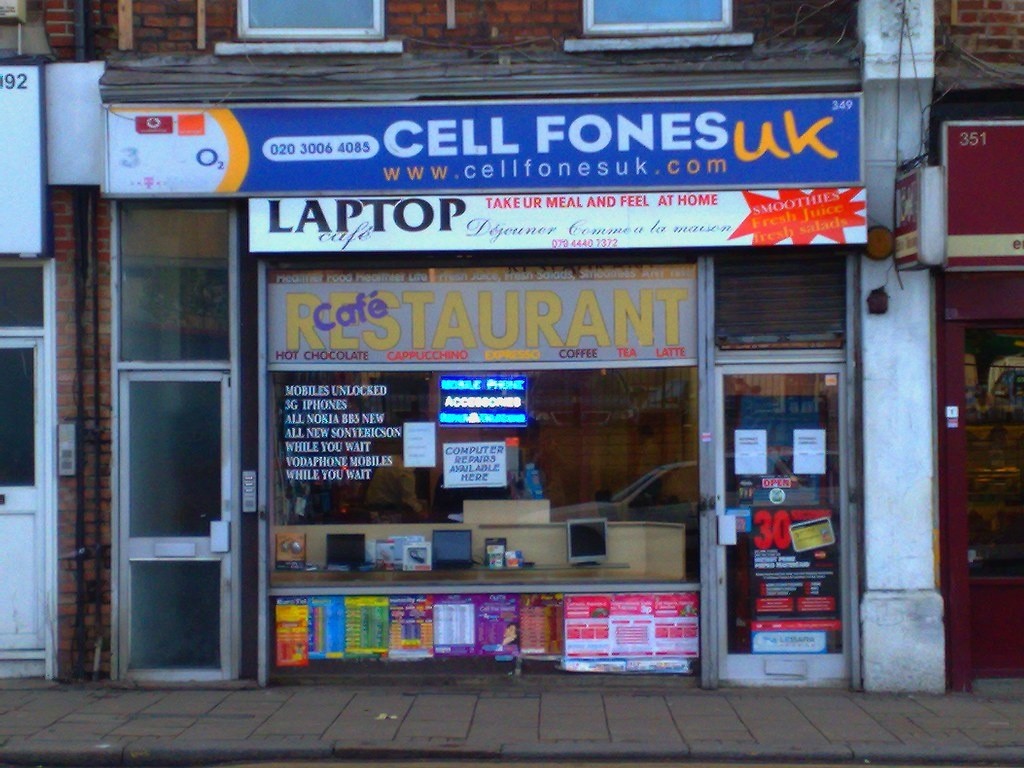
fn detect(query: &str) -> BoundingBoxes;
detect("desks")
[271,522,686,588]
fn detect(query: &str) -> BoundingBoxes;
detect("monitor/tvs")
[432,530,472,569]
[326,534,365,567]
[437,375,529,428]
[567,517,608,565]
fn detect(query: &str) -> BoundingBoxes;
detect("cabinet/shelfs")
[962,389,1024,578]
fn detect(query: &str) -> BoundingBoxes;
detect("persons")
[432,470,515,524]
[363,455,429,523]
[503,622,519,646]
